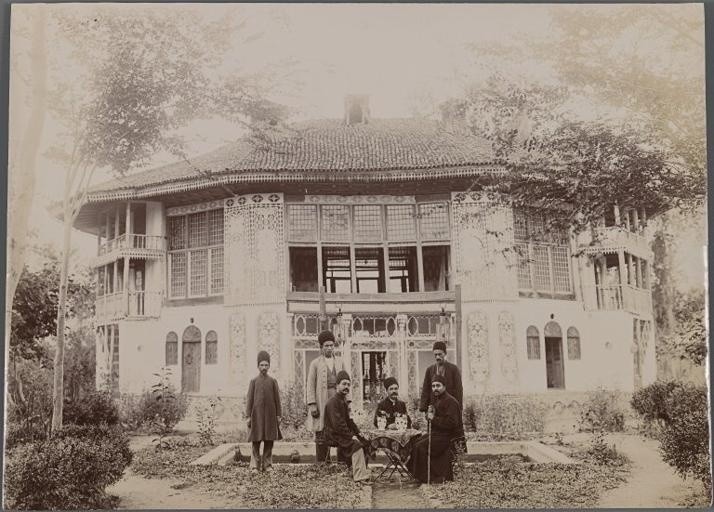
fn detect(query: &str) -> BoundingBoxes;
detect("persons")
[323,371,375,486]
[419,341,464,416]
[373,378,415,473]
[306,330,346,469]
[244,350,284,476]
[410,375,468,484]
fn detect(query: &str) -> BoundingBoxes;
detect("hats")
[337,371,350,384]
[432,341,447,354]
[383,377,399,388]
[430,374,448,386]
[317,331,335,345]
[256,350,270,367]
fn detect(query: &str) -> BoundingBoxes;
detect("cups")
[396,417,407,433]
[377,417,386,430]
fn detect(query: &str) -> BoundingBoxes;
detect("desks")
[358,429,428,482]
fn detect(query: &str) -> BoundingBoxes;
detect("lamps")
[439,307,446,325]
[336,308,343,325]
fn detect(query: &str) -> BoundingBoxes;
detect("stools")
[318,440,346,466]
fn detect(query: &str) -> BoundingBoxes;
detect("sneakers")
[251,468,258,475]
[263,465,273,473]
[359,479,375,485]
[399,475,420,489]
